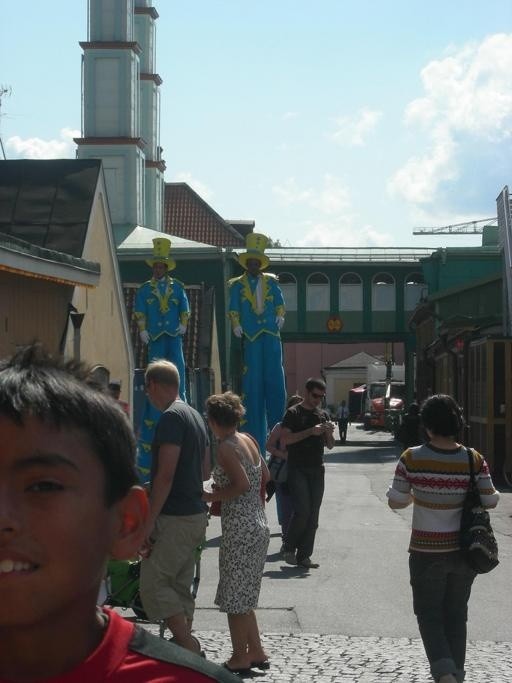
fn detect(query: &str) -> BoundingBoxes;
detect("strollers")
[98,508,202,620]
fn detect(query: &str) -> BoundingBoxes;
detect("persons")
[0,333,248,683]
[89,363,107,392]
[335,398,352,442]
[393,402,423,453]
[137,356,211,661]
[267,395,306,552]
[224,256,287,457]
[280,376,336,567]
[386,394,501,683]
[202,388,272,671]
[134,263,194,483]
[108,382,129,416]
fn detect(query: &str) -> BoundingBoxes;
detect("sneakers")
[280,545,320,568]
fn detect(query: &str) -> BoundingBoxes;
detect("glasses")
[311,391,325,399]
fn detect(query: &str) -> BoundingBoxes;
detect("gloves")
[233,326,244,339]
[139,330,150,344]
[175,322,187,335]
[275,315,285,329]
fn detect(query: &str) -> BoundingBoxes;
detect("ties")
[342,408,344,417]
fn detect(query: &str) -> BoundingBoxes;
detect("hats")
[238,233,270,270]
[144,238,177,273]
[265,479,276,502]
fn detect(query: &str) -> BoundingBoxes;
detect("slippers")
[223,662,270,675]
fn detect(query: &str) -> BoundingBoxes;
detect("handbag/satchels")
[460,477,499,574]
[266,457,286,482]
[211,473,266,516]
